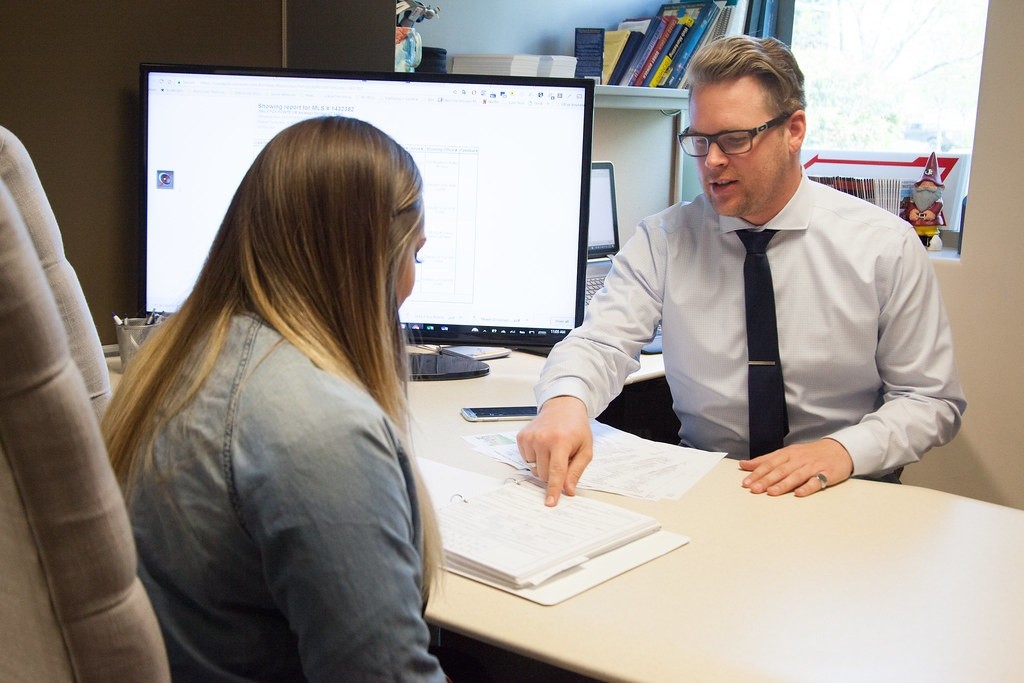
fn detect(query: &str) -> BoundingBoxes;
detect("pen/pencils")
[111,308,165,348]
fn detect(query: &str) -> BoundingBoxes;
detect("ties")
[734,230,791,461]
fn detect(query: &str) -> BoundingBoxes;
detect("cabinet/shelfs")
[279,1,794,304]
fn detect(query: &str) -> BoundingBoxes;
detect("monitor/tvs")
[138,62,595,380]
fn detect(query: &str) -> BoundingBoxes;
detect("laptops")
[584,160,620,313]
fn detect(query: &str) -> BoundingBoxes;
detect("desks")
[101,339,1024,683]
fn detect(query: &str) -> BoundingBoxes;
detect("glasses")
[678,113,787,158]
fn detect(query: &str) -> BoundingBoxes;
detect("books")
[409,412,732,606]
[807,175,917,218]
[451,0,782,89]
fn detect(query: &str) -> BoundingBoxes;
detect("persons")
[516,32,968,506]
[98,117,453,683]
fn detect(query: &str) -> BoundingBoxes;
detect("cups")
[116,318,163,372]
[395,27,421,72]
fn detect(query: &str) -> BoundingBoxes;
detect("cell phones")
[461,406,539,422]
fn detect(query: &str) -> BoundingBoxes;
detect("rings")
[811,472,829,490]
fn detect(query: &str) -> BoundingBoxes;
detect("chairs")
[0,125,173,682]
